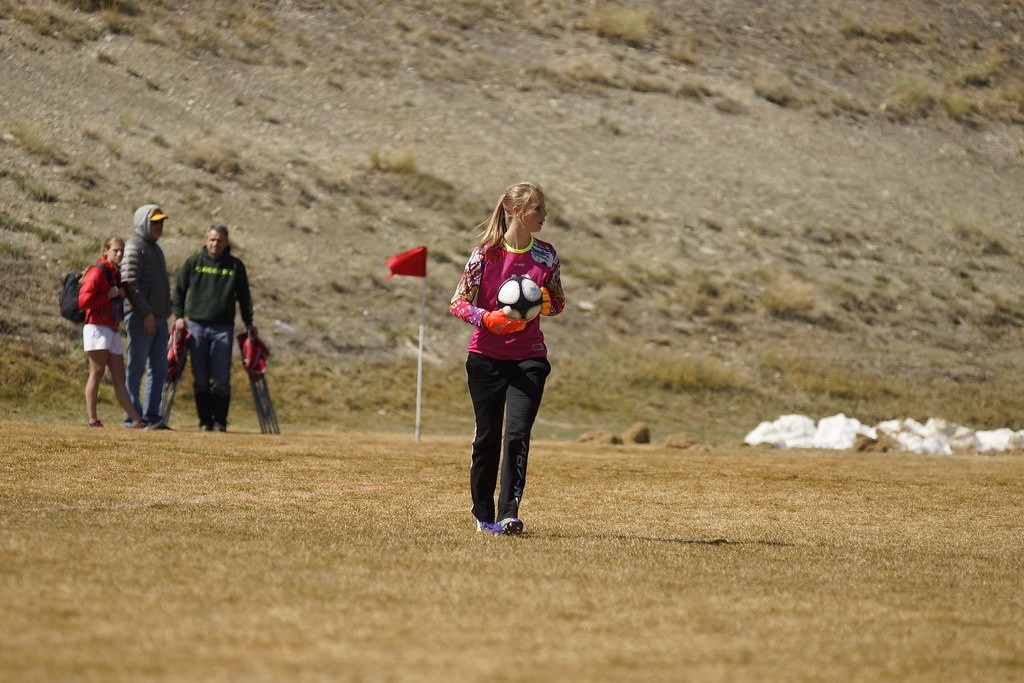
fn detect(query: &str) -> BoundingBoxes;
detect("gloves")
[540,286,559,316]
[482,305,527,335]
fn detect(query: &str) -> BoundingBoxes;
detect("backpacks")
[58,271,87,325]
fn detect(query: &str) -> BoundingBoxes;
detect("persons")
[119,203,175,431]
[78,235,164,430]
[449,181,567,534]
[172,224,257,434]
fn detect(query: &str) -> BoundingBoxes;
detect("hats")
[150,208,169,222]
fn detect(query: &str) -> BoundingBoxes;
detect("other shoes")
[198,423,212,431]
[122,417,135,428]
[213,422,226,432]
[151,422,177,431]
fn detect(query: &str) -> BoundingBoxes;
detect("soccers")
[497,276,545,323]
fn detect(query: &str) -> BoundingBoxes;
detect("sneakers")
[87,418,105,428]
[476,518,495,532]
[132,416,164,430]
[493,517,524,536]
